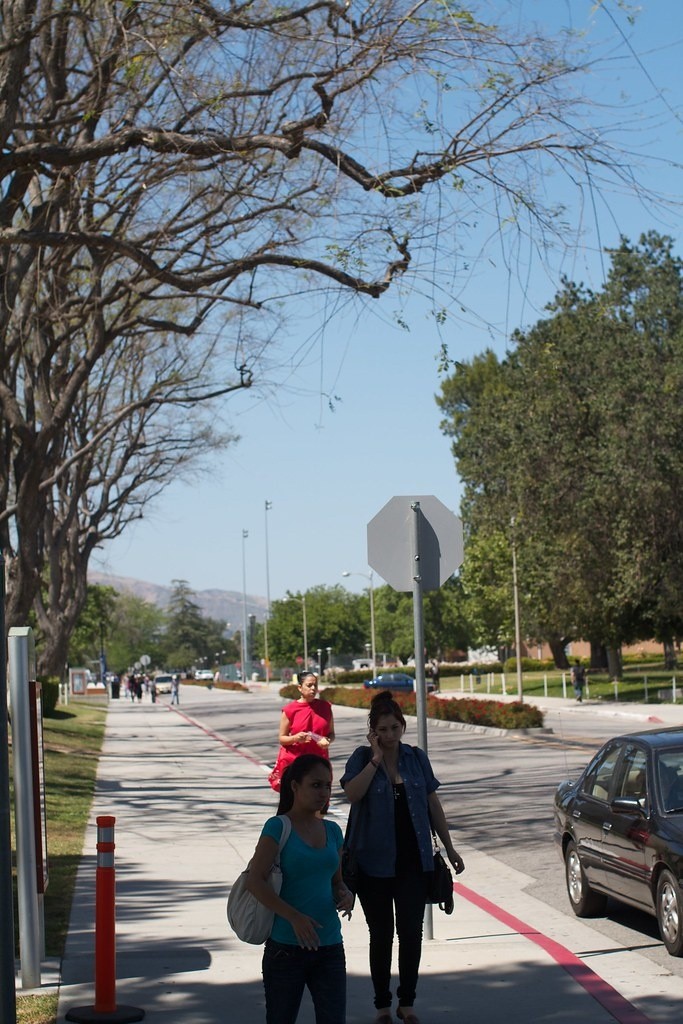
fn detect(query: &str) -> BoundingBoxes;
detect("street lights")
[265,499,278,683]
[283,598,308,671]
[241,528,248,683]
[342,569,377,679]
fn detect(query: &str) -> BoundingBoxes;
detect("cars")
[195,670,214,681]
[154,675,174,694]
[362,674,436,694]
[553,726,682,957]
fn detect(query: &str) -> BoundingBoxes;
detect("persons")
[149,673,157,703]
[123,666,148,702]
[569,658,589,704]
[340,691,466,1024]
[268,671,335,820]
[428,657,442,692]
[245,753,357,1024]
[170,674,180,706]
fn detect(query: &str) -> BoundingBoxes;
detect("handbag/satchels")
[424,851,454,915]
[226,814,292,945]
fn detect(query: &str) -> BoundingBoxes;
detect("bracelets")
[369,757,380,768]
[345,890,354,899]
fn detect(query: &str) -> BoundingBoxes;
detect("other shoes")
[395,1006,420,1024]
[377,1015,393,1024]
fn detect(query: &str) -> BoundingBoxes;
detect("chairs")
[626,771,644,795]
